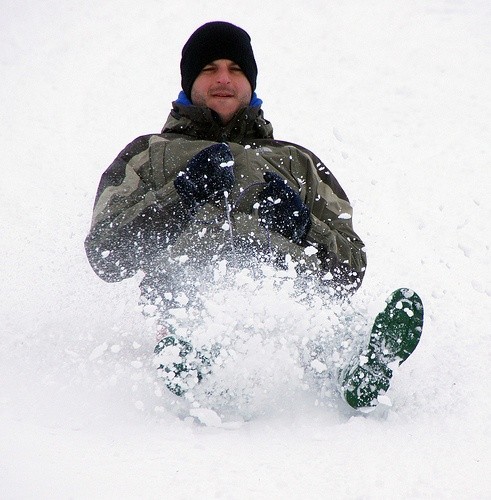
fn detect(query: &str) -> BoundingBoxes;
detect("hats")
[180,22,258,105]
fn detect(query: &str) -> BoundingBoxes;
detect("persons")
[84,22,424,428]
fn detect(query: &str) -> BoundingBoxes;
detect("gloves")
[257,171,312,246]
[174,142,235,210]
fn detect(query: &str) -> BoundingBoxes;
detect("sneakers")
[338,288,424,413]
[154,337,214,397]
